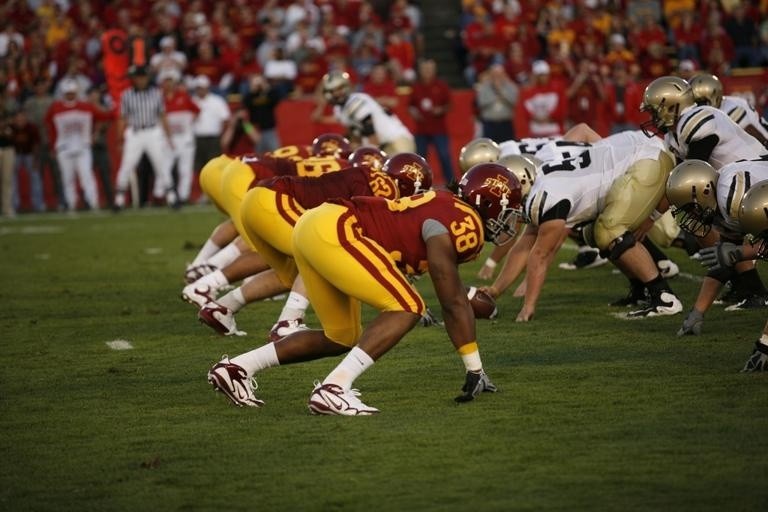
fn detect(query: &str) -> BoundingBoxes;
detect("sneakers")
[180,278,217,310]
[657,259,680,279]
[712,283,744,305]
[196,301,248,337]
[723,292,768,313]
[182,262,218,285]
[685,240,702,259]
[207,354,266,408]
[608,283,652,306]
[267,317,310,341]
[558,243,609,270]
[615,288,684,320]
[307,379,380,417]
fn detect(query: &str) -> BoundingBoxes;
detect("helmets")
[665,159,720,239]
[381,151,434,199]
[688,71,725,110]
[459,136,501,172]
[498,154,537,200]
[737,179,768,262]
[637,75,695,139]
[348,146,389,173]
[312,133,354,159]
[321,69,351,105]
[59,78,79,107]
[457,162,533,248]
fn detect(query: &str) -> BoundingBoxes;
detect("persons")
[12,109,47,217]
[240,73,294,152]
[44,77,118,211]
[220,108,262,157]
[496,150,680,297]
[632,75,768,310]
[738,179,768,374]
[407,55,455,185]
[463,0,652,144]
[0,107,17,217]
[112,64,173,212]
[1,1,423,106]
[457,129,683,322]
[688,73,768,149]
[191,75,236,205]
[180,146,389,311]
[665,159,768,338]
[182,133,353,283]
[197,152,434,342]
[206,162,522,417]
[459,137,610,279]
[310,70,418,158]
[652,0,768,76]
[81,86,113,210]
[152,70,200,207]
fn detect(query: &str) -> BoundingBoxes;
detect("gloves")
[675,308,706,337]
[453,366,498,404]
[421,305,442,327]
[697,240,742,273]
[739,333,768,373]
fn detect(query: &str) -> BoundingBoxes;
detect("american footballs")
[465,286,498,319]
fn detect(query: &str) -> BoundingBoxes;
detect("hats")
[531,59,551,75]
[194,75,210,88]
[155,67,180,85]
[488,52,505,64]
[126,65,144,77]
